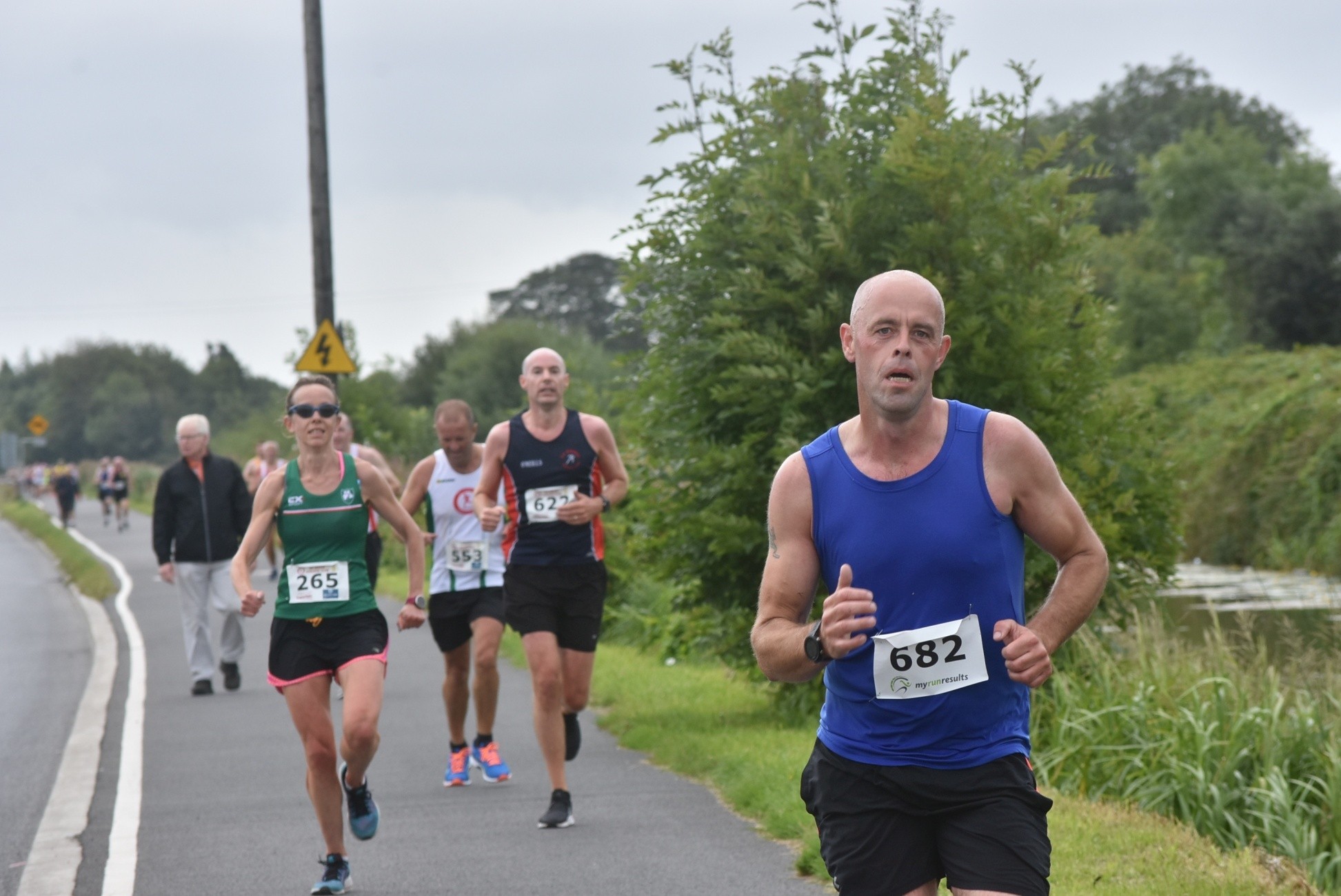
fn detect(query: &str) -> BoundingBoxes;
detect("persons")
[10,449,134,534]
[152,415,257,697]
[240,411,401,605]
[232,376,427,895]
[395,398,517,790]
[751,270,1109,896]
[474,347,630,828]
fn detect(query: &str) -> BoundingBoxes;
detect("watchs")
[406,595,427,610]
[803,619,828,664]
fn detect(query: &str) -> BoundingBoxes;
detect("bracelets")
[597,493,610,512]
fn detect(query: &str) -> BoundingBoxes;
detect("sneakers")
[564,714,581,761]
[310,852,353,896]
[339,762,379,840]
[443,742,512,786]
[537,790,575,829]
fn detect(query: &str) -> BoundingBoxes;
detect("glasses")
[284,403,337,419]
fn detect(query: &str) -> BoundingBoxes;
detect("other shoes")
[192,680,213,694]
[221,663,240,689]
